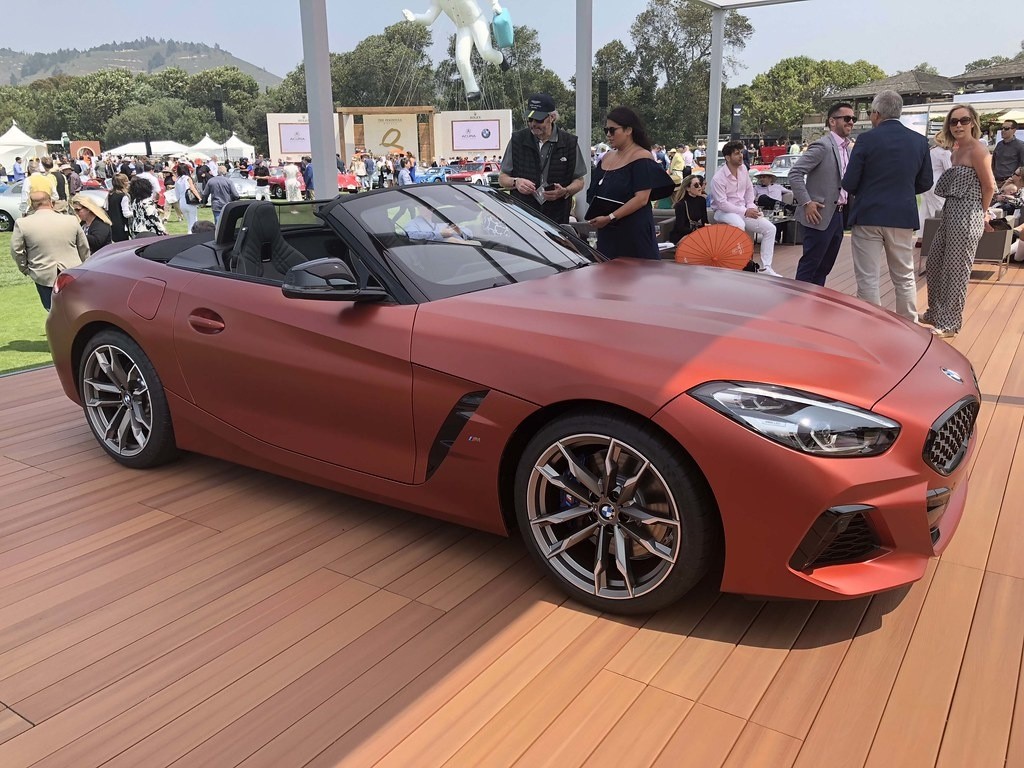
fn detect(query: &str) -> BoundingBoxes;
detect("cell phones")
[544,184,556,192]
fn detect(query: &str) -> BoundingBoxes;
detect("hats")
[523,92,555,120]
[667,149,676,155]
[286,157,293,162]
[678,144,686,148]
[754,171,777,182]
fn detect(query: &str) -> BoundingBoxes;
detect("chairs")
[484,167,490,172]
[916,209,1015,281]
[227,201,309,282]
[349,206,409,289]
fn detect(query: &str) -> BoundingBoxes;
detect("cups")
[655,226,660,237]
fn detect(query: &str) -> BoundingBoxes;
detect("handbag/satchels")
[185,188,203,204]
[163,188,179,203]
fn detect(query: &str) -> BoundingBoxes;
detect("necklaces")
[598,146,630,186]
[422,216,437,231]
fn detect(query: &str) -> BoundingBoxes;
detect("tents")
[0,120,255,180]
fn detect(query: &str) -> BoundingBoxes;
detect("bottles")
[589,232,597,250]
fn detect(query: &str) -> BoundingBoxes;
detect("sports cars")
[44,180,983,619]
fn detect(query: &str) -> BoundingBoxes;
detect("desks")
[753,216,798,245]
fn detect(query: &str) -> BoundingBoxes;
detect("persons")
[584,107,676,260]
[336,148,502,194]
[0,153,314,312]
[498,93,588,227]
[710,140,783,277]
[990,119,1024,264]
[590,142,607,169]
[918,105,994,338]
[392,158,416,224]
[402,200,475,243]
[842,89,934,323]
[754,170,797,216]
[670,175,759,274]
[402,0,509,102]
[787,101,858,286]
[743,140,809,171]
[650,139,706,182]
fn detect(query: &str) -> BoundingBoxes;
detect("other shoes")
[915,242,924,247]
[939,330,955,338]
[756,267,783,278]
[918,314,933,323]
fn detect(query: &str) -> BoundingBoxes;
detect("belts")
[834,205,847,211]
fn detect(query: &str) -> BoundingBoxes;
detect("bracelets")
[565,188,570,199]
[512,178,518,187]
[608,213,617,222]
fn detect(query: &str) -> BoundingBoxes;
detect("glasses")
[529,114,550,123]
[1001,126,1012,130]
[949,116,973,126]
[688,183,702,188]
[1013,173,1020,177]
[74,207,83,212]
[867,111,880,116]
[604,126,627,135]
[833,115,858,123]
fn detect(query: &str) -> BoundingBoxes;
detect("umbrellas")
[675,222,754,270]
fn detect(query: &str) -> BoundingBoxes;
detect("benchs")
[652,209,751,261]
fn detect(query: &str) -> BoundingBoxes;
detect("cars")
[751,154,807,189]
[446,161,503,185]
[415,164,464,183]
[266,165,308,198]
[0,178,31,231]
